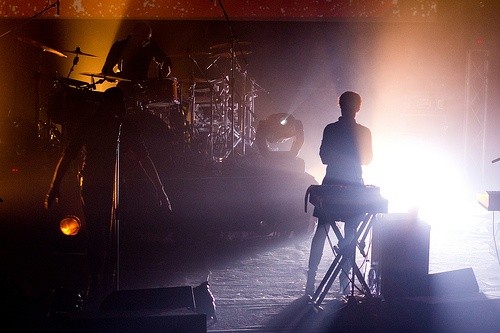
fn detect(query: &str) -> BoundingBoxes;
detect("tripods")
[184,2,271,162]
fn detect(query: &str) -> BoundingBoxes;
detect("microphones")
[55,0,60,17]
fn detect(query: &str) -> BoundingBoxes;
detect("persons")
[102,22,171,91]
[306,91,373,297]
[45,87,148,301]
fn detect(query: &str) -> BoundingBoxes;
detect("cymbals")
[79,72,131,82]
[16,36,67,58]
[61,49,97,58]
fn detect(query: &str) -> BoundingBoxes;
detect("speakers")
[370,219,430,296]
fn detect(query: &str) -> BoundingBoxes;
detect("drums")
[143,75,180,107]
